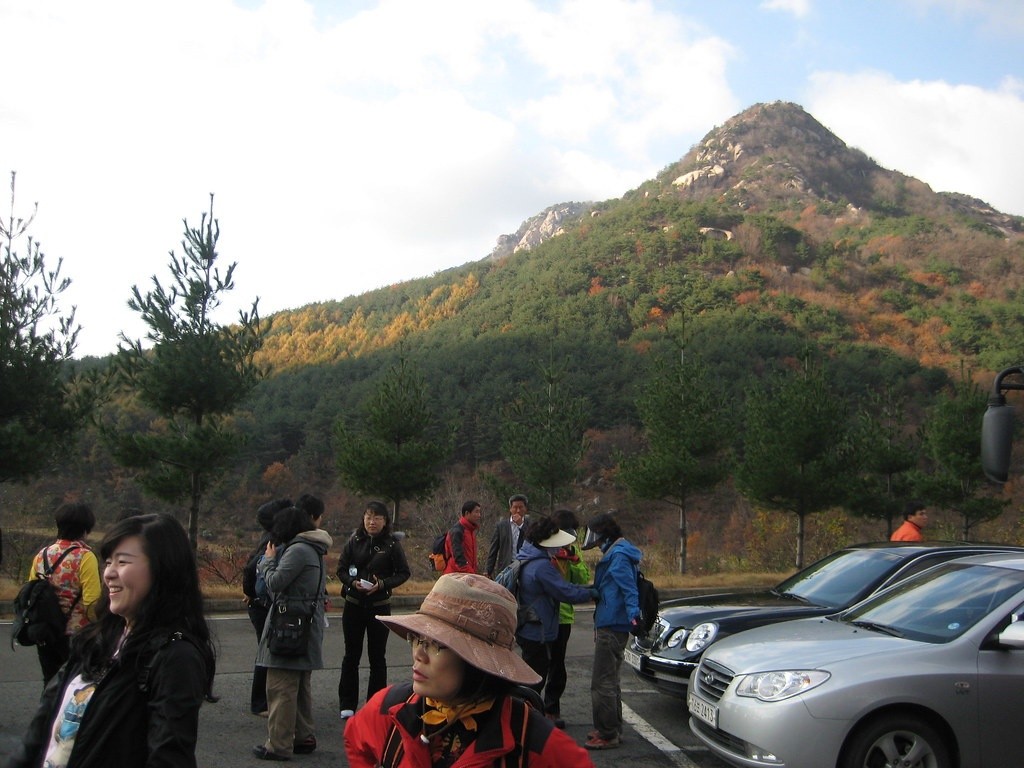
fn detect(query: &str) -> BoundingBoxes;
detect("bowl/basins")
[360,579,373,589]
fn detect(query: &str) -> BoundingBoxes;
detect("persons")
[890,499,928,541]
[487,494,531,580]
[343,573,596,768]
[29,502,102,689]
[581,510,644,750]
[442,501,481,574]
[336,502,411,718]
[6,511,222,768]
[514,510,598,729]
[243,494,333,760]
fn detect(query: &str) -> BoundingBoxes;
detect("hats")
[581,525,605,550]
[537,529,576,548]
[375,572,543,685]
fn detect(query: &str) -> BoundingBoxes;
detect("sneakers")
[584,729,623,750]
[293,736,316,754]
[340,710,354,719]
[252,745,289,761]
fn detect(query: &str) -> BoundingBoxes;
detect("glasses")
[407,632,448,656]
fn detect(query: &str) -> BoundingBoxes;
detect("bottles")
[349,565,357,579]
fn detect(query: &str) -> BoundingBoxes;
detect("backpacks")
[9,546,83,651]
[494,558,542,632]
[429,533,450,572]
[630,571,660,640]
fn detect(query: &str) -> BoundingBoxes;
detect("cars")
[620,539,1024,701]
[688,550,1024,768]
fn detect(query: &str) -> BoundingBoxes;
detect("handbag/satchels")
[340,581,350,598]
[265,601,313,657]
